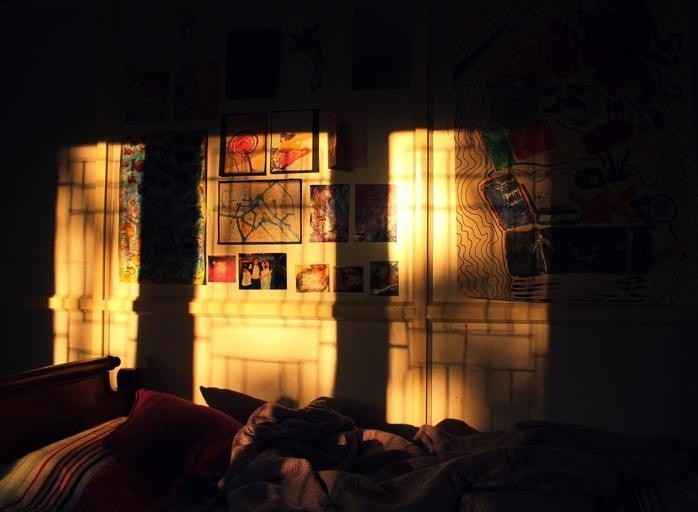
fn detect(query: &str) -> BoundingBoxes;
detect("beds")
[0,356,698,512]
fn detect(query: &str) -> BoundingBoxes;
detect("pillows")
[102,385,267,488]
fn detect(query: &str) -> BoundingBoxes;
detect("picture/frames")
[207,107,320,245]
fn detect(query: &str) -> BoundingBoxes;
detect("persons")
[241,258,273,288]
